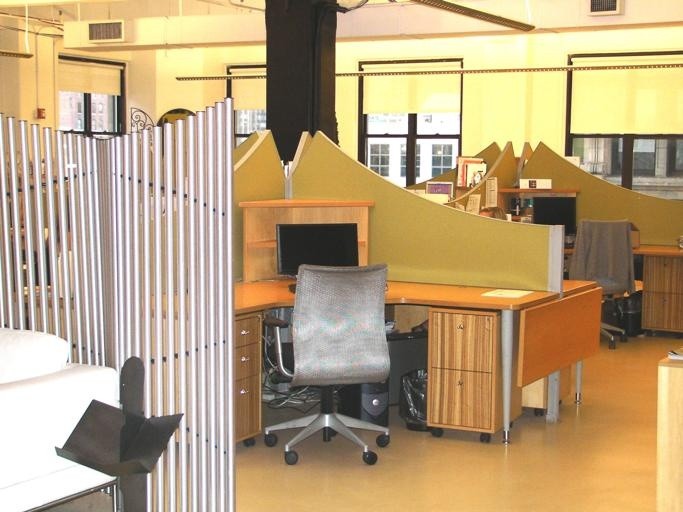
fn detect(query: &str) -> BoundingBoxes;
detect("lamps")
[0,5,33,58]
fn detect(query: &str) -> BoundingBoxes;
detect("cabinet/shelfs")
[641,255,683,337]
[232,311,265,446]
[426,306,523,441]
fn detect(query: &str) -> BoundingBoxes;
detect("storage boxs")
[629,222,640,249]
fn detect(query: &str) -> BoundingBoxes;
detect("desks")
[232,278,603,447]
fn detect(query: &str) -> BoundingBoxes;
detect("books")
[667,347,683,361]
[455,155,487,188]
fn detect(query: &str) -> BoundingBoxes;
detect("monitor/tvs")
[425,181,453,202]
[276,223,358,293]
[532,195,578,249]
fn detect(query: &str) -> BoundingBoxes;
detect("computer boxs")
[338,373,389,427]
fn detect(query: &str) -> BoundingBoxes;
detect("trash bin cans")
[401,368,427,431]
[615,296,643,336]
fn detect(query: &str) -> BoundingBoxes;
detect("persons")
[480,208,508,219]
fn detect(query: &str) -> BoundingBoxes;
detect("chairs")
[569,221,643,349]
[264,262,391,465]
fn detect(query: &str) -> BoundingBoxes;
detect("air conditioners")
[63,19,125,48]
